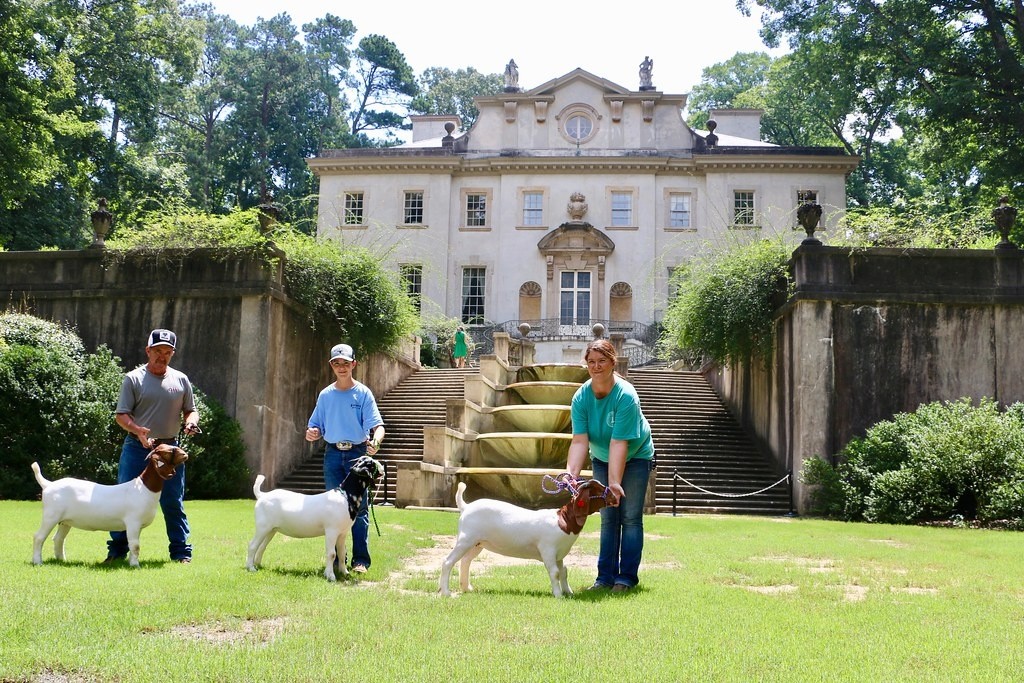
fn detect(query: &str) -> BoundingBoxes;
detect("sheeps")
[247,456,385,583]
[30,443,189,567]
[439,479,618,598]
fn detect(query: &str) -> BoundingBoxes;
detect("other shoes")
[179,557,190,564]
[588,582,613,590]
[611,584,628,591]
[104,556,125,563]
[352,564,368,573]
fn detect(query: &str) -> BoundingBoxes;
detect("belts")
[129,432,175,444]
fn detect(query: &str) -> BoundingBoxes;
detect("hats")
[328,344,356,361]
[148,329,176,348]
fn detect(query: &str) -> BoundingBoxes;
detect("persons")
[305,344,385,574]
[562,339,655,593]
[452,327,474,368]
[103,329,200,564]
